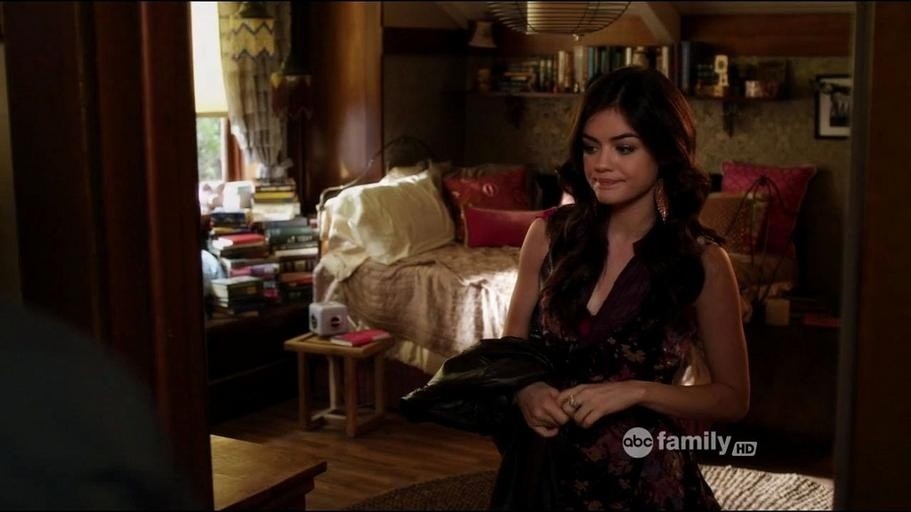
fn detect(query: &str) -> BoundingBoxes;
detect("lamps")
[486,0,631,41]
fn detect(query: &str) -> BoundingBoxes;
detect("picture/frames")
[815,71,850,141]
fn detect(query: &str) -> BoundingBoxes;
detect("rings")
[565,393,578,408]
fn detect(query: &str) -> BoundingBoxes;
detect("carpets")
[326,448,835,512]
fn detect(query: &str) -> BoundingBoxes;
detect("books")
[497,38,789,97]
[330,328,394,347]
[205,177,319,316]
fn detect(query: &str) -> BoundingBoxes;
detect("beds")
[310,128,788,422]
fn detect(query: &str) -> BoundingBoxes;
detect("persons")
[500,61,755,511]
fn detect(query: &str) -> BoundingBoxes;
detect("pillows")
[696,157,818,256]
[321,155,558,288]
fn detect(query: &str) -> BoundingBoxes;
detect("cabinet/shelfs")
[203,300,309,413]
